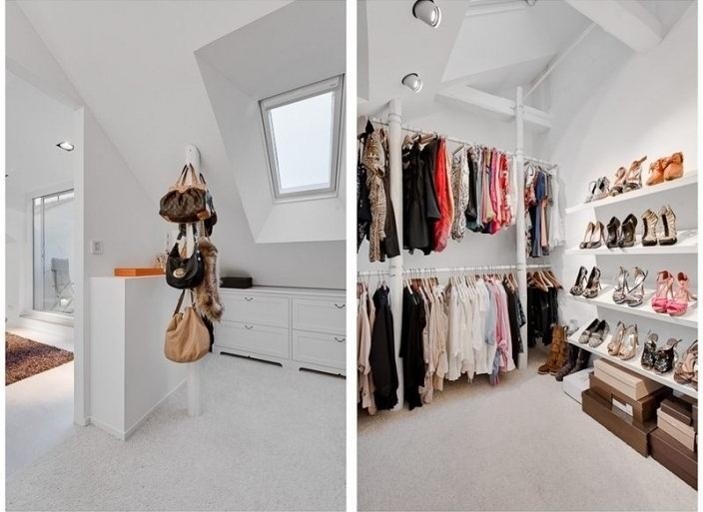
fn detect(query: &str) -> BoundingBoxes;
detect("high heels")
[652,271,675,313]
[580,221,605,248]
[570,265,589,295]
[586,265,602,298]
[608,322,640,360]
[628,266,648,307]
[613,268,629,303]
[667,271,691,315]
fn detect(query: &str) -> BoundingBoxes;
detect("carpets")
[5,330,74,386]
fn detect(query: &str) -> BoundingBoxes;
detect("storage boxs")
[580,357,698,492]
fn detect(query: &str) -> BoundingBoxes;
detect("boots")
[539,325,591,380]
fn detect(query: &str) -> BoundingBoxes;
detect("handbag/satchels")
[166,232,204,288]
[159,163,218,237]
[165,290,210,362]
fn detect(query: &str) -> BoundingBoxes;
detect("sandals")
[606,215,638,247]
[641,207,677,245]
[585,154,683,202]
[642,334,698,390]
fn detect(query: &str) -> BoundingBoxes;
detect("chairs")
[48,258,76,313]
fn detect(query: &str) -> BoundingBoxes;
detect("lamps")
[401,72,423,93]
[411,0,441,28]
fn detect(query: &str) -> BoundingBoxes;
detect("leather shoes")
[579,318,608,347]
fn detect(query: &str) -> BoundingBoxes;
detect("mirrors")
[31,188,74,315]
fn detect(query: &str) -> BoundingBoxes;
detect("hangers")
[358,261,564,304]
[366,115,557,179]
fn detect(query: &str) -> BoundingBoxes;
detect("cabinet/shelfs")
[562,174,697,405]
[292,286,346,379]
[210,286,292,370]
